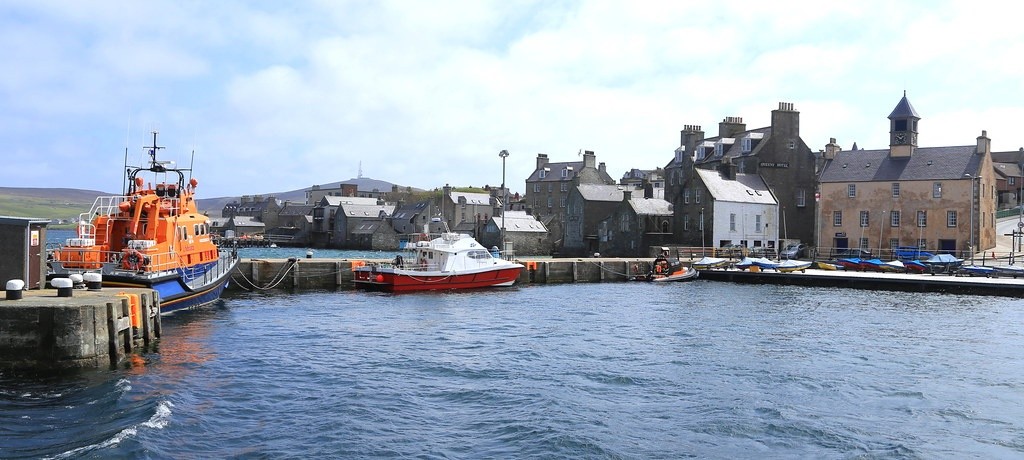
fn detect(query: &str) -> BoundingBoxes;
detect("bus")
[894,246,920,254]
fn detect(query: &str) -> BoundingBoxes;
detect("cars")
[780,243,808,259]
[838,248,876,255]
[397,233,430,249]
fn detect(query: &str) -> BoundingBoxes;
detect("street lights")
[498,149,508,250]
[701,207,706,258]
[782,205,789,240]
[917,209,925,257]
[964,171,983,265]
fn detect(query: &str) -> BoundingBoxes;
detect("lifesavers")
[421,259,427,270]
[417,233,428,241]
[123,250,143,270]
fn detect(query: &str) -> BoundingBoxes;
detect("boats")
[50,130,241,316]
[627,257,699,286]
[694,252,1024,279]
[353,232,524,298]
